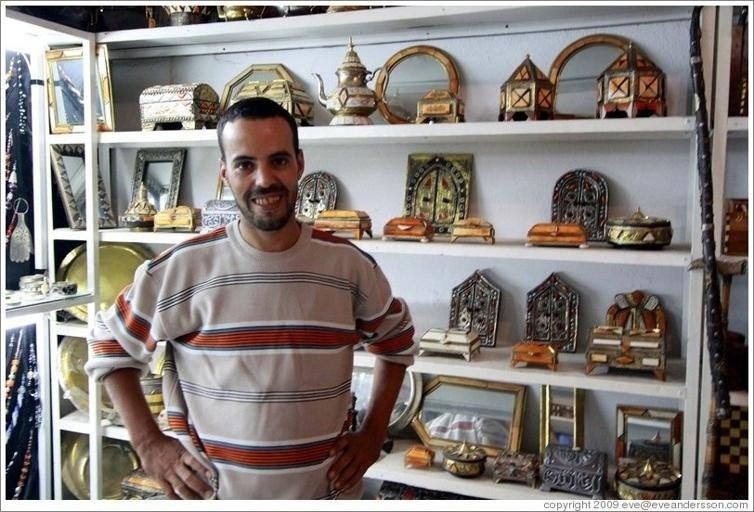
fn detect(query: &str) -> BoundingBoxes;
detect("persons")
[85,95,414,499]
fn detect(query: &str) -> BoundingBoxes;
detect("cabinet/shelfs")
[47,6,718,500]
[695,6,748,499]
[6,9,100,500]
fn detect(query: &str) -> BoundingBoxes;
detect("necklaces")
[6,54,28,246]
[4,329,41,499]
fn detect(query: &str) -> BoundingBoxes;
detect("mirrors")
[46,44,294,231]
[349,366,683,471]
[548,33,631,119]
[375,44,460,125]
